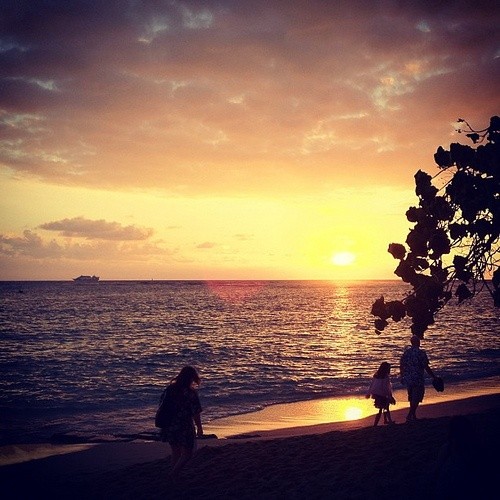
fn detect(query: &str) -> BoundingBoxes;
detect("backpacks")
[154,385,191,428]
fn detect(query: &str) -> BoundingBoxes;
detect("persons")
[367,361,398,427]
[158,364,204,477]
[400,336,437,422]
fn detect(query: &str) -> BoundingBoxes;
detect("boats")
[73,275,99,286]
